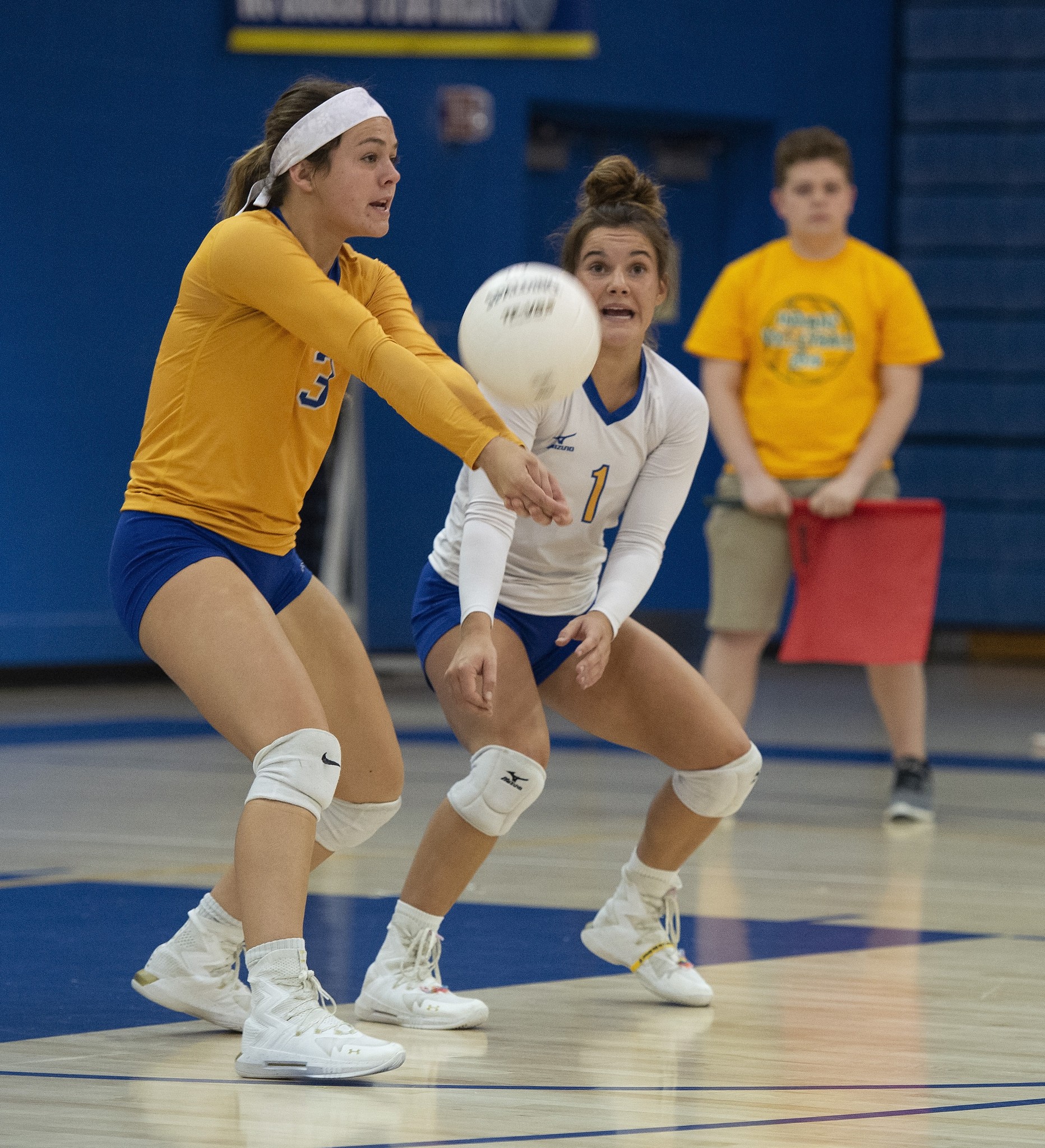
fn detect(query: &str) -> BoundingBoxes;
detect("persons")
[104,79,572,1080]
[354,155,761,1032]
[682,125,944,821]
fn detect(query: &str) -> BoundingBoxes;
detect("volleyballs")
[457,259,603,409]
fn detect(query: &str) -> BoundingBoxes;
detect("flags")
[784,494,942,669]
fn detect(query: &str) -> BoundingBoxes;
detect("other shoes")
[886,759,935,820]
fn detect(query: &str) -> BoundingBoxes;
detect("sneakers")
[353,913,487,1028]
[131,909,252,1031]
[583,1008,710,1102]
[235,948,407,1079]
[578,866,714,1010]
